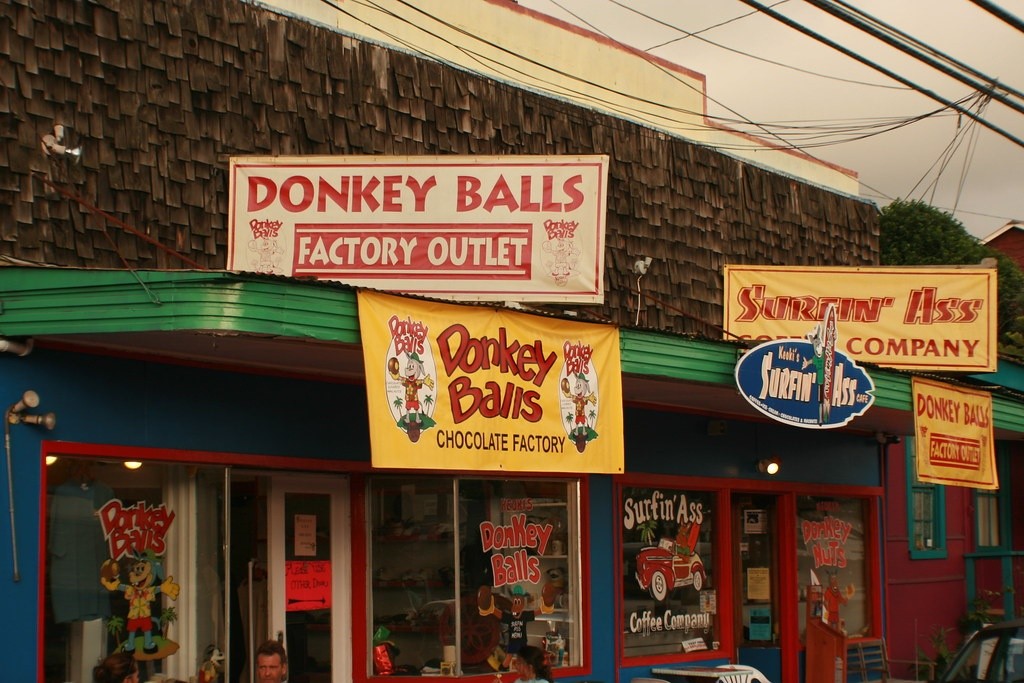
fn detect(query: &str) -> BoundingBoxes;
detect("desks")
[652,665,753,682]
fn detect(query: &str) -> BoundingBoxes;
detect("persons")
[93,653,140,683]
[515,645,550,683]
[256,640,288,683]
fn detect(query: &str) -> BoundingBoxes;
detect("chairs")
[630,677,672,683]
[715,664,772,683]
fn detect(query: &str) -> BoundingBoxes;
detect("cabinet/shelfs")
[500,477,580,666]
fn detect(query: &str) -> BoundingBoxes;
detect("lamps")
[876,431,901,445]
[11,389,41,412]
[41,124,83,166]
[759,455,781,474]
[20,411,55,430]
[634,255,653,275]
